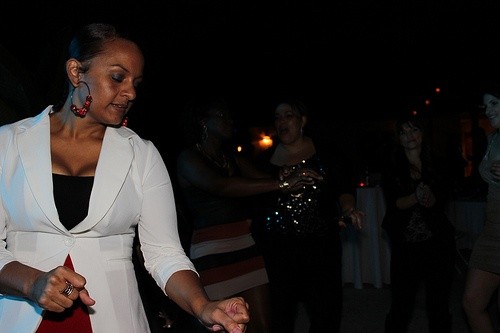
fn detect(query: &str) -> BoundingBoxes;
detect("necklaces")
[195,142,230,169]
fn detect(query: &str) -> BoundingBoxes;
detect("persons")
[462,80,500,332]
[132,93,326,333]
[379,114,466,333]
[250,97,368,332]
[3,17,250,333]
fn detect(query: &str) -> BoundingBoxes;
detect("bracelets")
[277,180,293,194]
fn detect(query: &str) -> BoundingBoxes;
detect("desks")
[342,185,392,291]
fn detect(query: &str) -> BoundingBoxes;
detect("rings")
[60,279,75,296]
